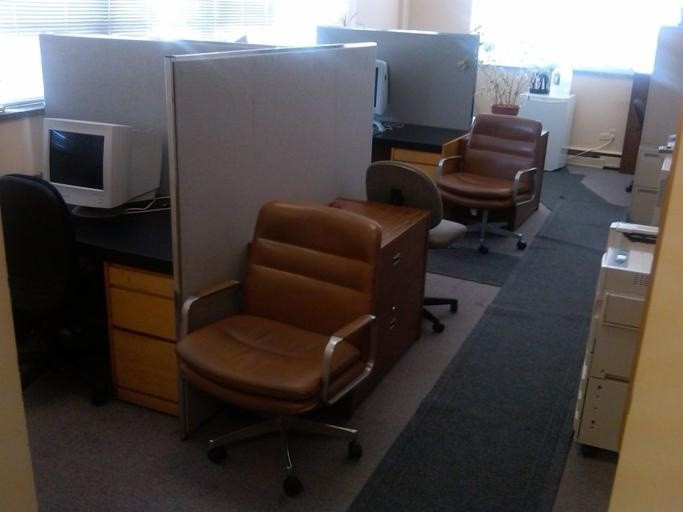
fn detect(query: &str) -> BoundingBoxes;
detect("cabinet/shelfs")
[324,195,431,419]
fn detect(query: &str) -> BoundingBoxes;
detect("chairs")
[364,161,467,337]
[0,174,108,407]
[623,97,646,194]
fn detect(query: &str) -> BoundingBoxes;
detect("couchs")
[433,112,541,254]
[175,199,382,497]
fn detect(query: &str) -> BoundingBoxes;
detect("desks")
[66,200,177,420]
[372,119,468,218]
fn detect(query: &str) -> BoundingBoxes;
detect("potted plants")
[454,22,542,115]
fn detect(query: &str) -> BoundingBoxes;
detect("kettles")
[529,72,548,94]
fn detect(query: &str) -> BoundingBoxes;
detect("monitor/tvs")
[42,118,161,218]
[372,59,388,132]
